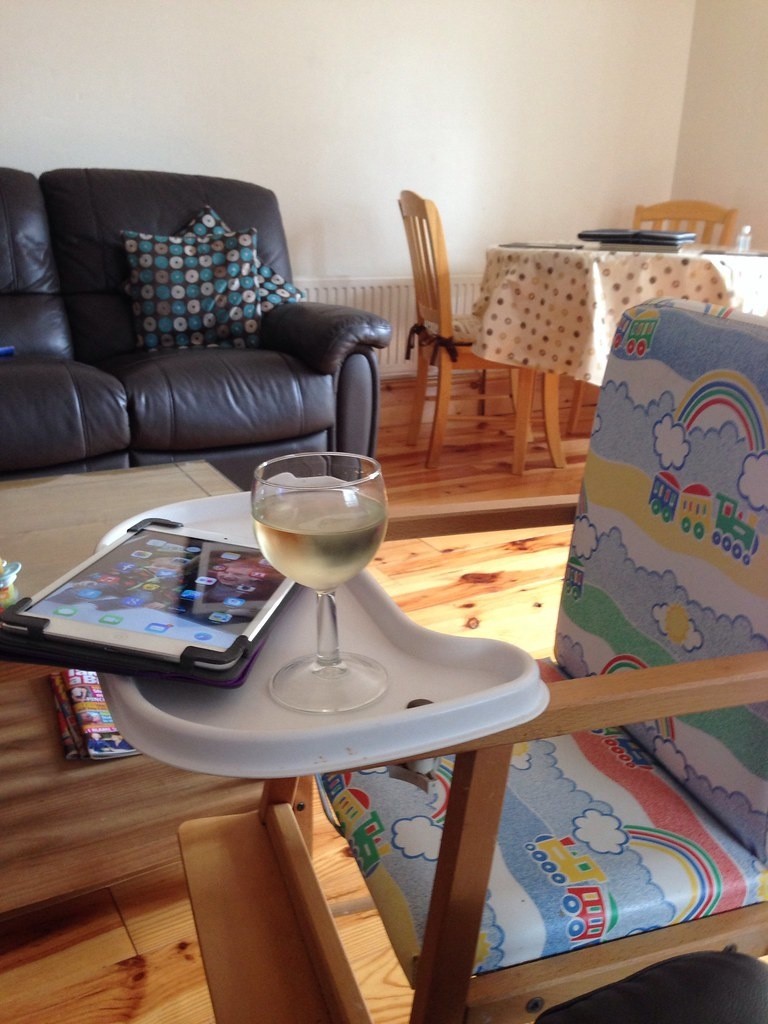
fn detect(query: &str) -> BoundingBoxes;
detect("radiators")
[294,278,512,378]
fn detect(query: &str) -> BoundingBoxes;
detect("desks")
[1,462,314,916]
[473,246,768,474]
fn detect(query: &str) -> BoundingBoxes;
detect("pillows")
[120,204,304,350]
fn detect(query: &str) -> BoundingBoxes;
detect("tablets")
[0,517,296,671]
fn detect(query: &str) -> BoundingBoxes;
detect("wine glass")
[250,452,389,714]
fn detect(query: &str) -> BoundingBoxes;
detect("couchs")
[0,166,392,492]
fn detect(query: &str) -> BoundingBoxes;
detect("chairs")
[398,189,572,469]
[633,197,738,246]
[94,297,768,1024]
